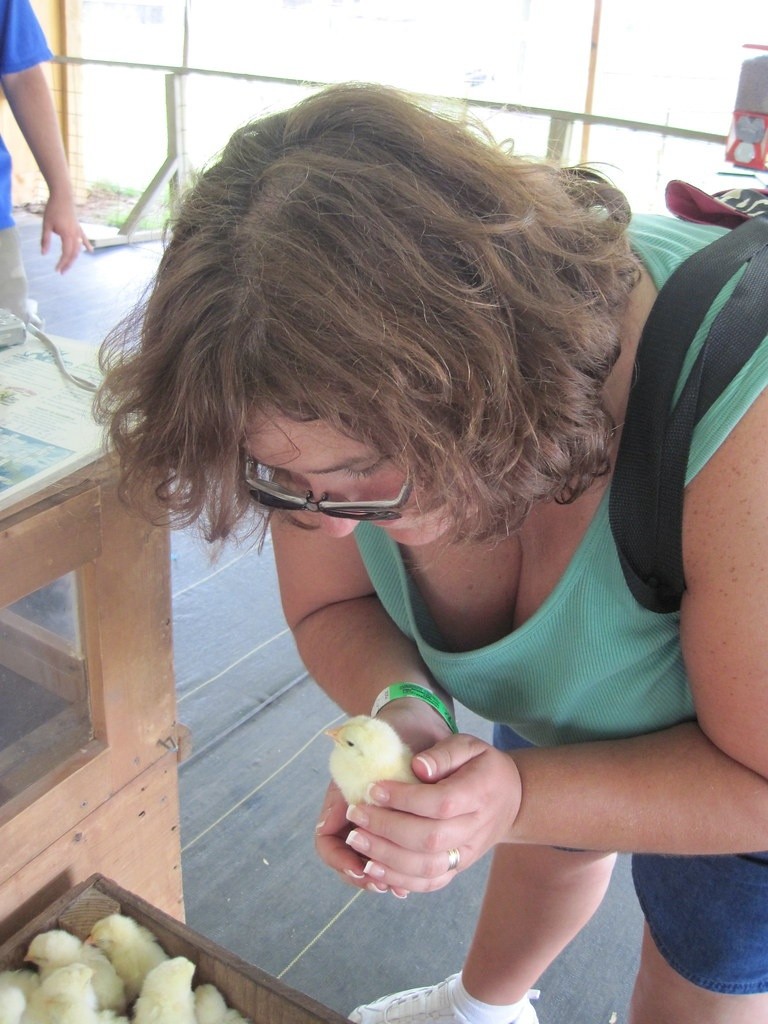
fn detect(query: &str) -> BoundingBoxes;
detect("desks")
[0,329,186,927]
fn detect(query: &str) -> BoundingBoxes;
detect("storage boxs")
[0,872,355,1024]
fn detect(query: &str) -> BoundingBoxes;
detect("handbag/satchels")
[665,180,768,228]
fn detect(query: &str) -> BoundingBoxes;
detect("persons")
[0,0,92,325]
[90,83,768,1023]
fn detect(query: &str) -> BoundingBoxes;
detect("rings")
[447,848,460,870]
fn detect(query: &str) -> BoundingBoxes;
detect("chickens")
[0,914,250,1024]
[322,715,423,807]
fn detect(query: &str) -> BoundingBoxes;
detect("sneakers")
[348,973,543,1024]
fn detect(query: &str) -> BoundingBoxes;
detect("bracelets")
[370,681,459,734]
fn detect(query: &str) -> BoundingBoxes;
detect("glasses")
[235,436,416,521]
